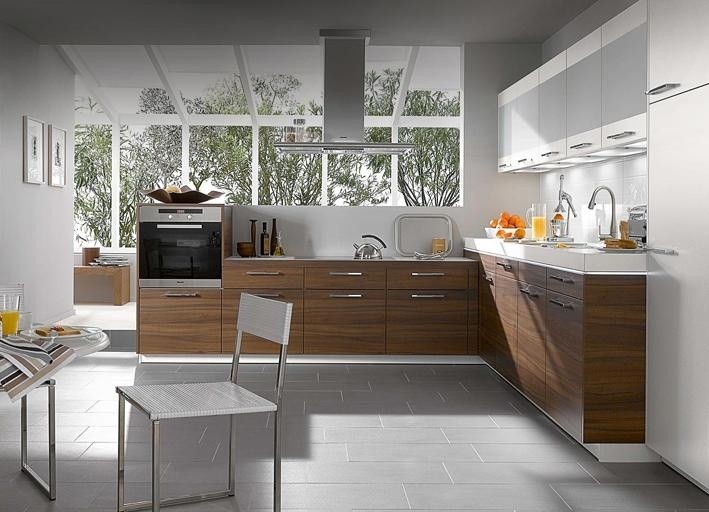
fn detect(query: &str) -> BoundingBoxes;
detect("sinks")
[524,242,597,250]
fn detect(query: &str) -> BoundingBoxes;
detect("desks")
[74,265,130,306]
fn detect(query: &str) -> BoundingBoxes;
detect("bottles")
[270,218,278,256]
[260,222,270,255]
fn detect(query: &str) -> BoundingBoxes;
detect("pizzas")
[605,237,638,249]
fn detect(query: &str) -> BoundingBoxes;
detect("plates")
[33,326,102,339]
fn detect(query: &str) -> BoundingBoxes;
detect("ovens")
[137,203,223,289]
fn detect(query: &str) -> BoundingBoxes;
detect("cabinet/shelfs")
[222,266,303,354]
[478,272,495,363]
[566,26,615,165]
[387,268,468,354]
[602,10,647,152]
[496,89,546,174]
[513,72,559,173]
[517,265,546,398]
[538,51,578,171]
[547,271,646,444]
[139,287,221,353]
[492,257,519,378]
[305,267,384,355]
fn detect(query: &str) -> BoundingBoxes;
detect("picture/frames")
[48,124,67,188]
[22,114,46,185]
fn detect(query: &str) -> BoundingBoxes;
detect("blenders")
[544,173,578,244]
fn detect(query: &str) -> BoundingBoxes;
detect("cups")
[0,294,20,338]
[294,118,305,142]
[284,126,295,144]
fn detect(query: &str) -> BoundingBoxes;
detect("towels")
[0,333,77,404]
[0,335,53,377]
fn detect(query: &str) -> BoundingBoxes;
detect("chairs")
[116,292,295,511]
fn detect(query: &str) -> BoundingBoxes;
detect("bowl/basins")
[237,242,254,257]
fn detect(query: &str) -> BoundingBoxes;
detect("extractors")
[275,28,416,152]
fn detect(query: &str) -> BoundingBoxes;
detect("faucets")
[586,184,619,241]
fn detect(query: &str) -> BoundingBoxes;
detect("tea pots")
[353,234,386,260]
